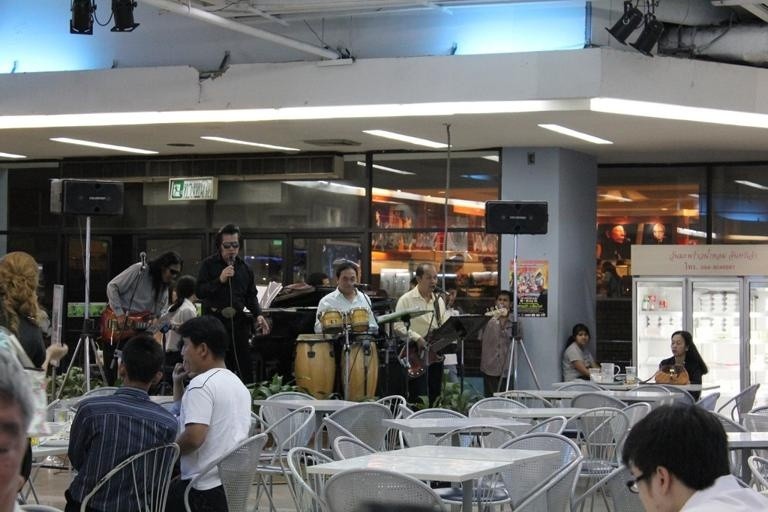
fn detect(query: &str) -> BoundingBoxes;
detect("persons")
[409,277,418,290]
[1,348,66,512]
[393,262,449,410]
[643,223,671,244]
[308,272,330,289]
[659,331,708,403]
[195,223,269,390]
[66,333,178,511]
[165,315,252,511]
[479,290,523,399]
[107,250,185,395]
[597,261,624,298]
[600,224,625,260]
[314,263,380,335]
[445,282,463,384]
[0,251,68,504]
[160,275,199,367]
[623,402,768,512]
[562,323,601,381]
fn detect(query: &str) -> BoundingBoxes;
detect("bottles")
[194,302,202,317]
[641,289,669,312]
[31,407,69,440]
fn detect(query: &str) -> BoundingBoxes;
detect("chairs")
[0,374,768,512]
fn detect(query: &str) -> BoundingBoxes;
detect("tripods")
[55,216,110,400]
[496,234,548,409]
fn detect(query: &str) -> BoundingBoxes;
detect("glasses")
[221,241,239,250]
[169,268,180,276]
[626,474,645,494]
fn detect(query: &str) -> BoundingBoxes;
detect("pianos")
[269,283,389,308]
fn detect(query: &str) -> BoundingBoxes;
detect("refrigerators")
[631,277,766,479]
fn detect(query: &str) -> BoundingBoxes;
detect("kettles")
[619,275,633,298]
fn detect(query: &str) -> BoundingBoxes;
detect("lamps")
[111,0,140,32]
[70,0,97,34]
[605,8,643,45]
[628,13,664,58]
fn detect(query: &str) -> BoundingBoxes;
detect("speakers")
[50,178,125,216]
[485,200,548,235]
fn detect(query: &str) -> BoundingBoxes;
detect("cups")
[588,367,600,382]
[598,363,620,383]
[623,366,636,383]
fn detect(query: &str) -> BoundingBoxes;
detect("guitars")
[100,305,179,345]
[397,305,509,378]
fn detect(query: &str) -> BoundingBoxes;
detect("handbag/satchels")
[655,365,690,385]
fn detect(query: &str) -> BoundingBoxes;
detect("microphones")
[354,283,368,288]
[228,255,235,266]
[139,251,147,274]
[434,286,451,296]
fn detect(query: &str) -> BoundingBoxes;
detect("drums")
[295,334,335,399]
[340,333,378,402]
[350,308,369,332]
[322,309,343,334]
